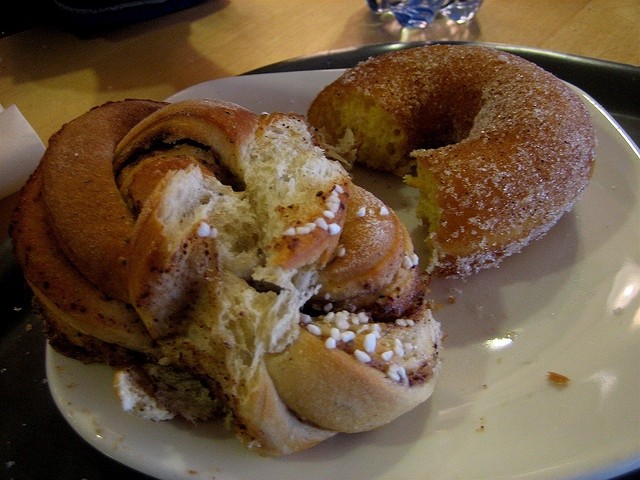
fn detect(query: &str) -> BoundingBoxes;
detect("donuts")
[307,45,594,280]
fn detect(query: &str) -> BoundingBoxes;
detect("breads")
[13,98,442,458]
